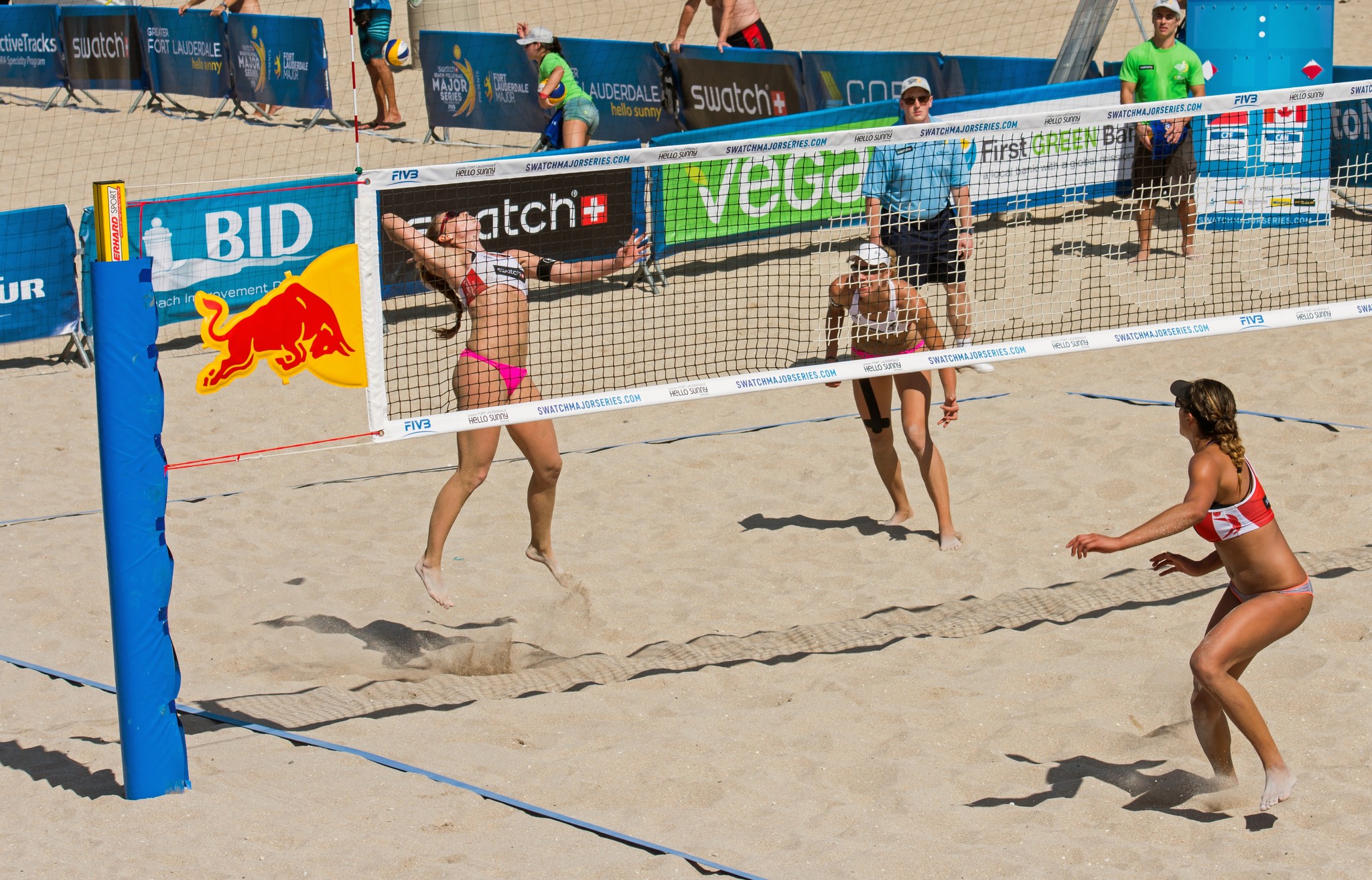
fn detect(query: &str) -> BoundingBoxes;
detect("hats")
[1170,380,1215,429]
[900,76,931,98]
[845,243,890,267]
[516,26,554,45]
[1152,0,1181,14]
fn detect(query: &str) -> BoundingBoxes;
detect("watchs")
[220,2,227,10]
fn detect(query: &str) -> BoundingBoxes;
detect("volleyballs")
[382,39,409,66]
[537,79,566,104]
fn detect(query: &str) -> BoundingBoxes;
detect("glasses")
[1175,397,1190,413]
[850,264,887,274]
[902,96,930,105]
[440,210,460,236]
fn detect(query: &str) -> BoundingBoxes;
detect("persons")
[824,243,960,553]
[1067,377,1314,819]
[379,209,652,610]
[1118,0,1206,264]
[671,0,775,55]
[353,0,405,131]
[861,75,995,375]
[178,0,284,117]
[517,22,600,151]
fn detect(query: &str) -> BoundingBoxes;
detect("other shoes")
[955,363,995,373]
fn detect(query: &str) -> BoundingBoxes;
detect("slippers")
[357,121,379,130]
[374,121,406,131]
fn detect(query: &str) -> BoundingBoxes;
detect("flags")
[1262,103,1307,128]
